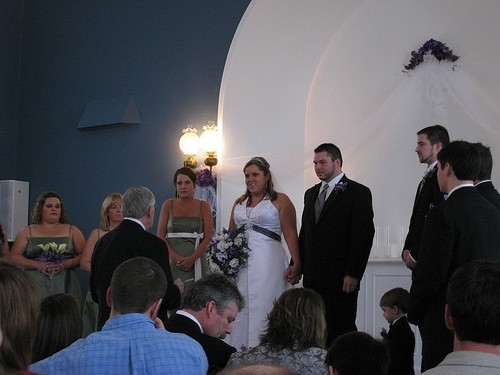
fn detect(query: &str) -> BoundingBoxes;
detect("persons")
[0,186,415,375]
[401,125,500,375]
[283,143,375,350]
[156,166,215,305]
[220,156,302,351]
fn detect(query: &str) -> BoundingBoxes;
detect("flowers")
[195,168,216,188]
[402,31,459,74]
[334,182,347,191]
[204,222,252,286]
[380,328,387,337]
[36,242,70,279]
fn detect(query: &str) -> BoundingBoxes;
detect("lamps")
[178,120,223,177]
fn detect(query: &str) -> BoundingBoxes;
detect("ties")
[314,184,329,223]
[419,172,429,191]
